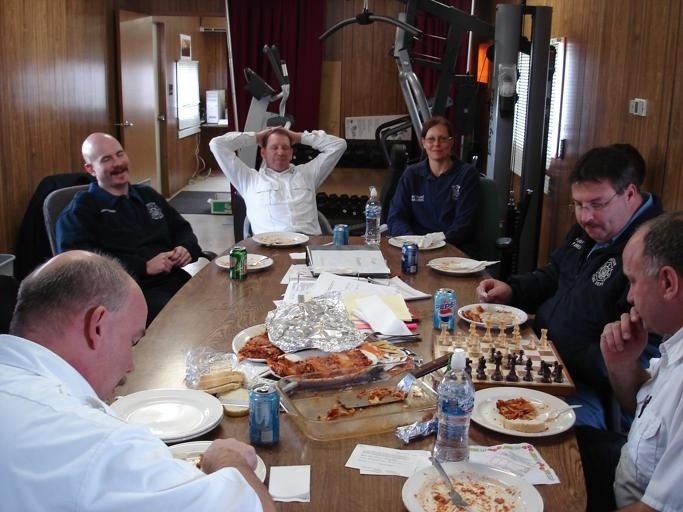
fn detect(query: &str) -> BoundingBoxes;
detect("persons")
[54,133,201,332]
[477,142,665,432]
[209,124,347,237]
[0,248,277,512]
[575,210,682,511]
[384,114,478,250]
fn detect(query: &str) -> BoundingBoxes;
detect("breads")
[198,369,248,416]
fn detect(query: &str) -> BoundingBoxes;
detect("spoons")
[535,405,582,422]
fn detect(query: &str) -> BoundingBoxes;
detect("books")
[305,244,392,276]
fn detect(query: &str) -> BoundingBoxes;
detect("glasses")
[569,190,620,212]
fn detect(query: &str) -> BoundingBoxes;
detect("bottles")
[107,388,266,491]
[432,350,475,462]
[365,188,382,247]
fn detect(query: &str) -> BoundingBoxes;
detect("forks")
[431,458,469,508]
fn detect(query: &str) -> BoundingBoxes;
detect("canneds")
[249,383,279,446]
[230,247,247,280]
[434,288,455,330]
[333,224,348,245]
[401,242,419,275]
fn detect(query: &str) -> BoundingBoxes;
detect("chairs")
[241,210,336,242]
[467,178,516,283]
[0,169,99,334]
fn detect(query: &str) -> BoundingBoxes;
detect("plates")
[269,348,378,387]
[233,323,284,361]
[215,232,309,272]
[276,366,438,442]
[402,464,545,512]
[470,386,575,436]
[458,305,529,327]
[388,234,488,274]
